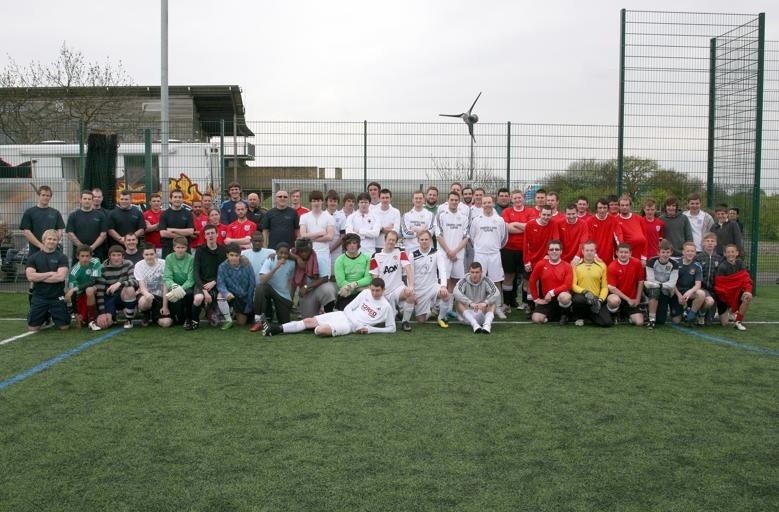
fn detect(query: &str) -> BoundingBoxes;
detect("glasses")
[277,196,288,198]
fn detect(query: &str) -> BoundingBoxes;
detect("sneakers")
[142,319,149,326]
[261,320,273,335]
[403,321,412,331]
[88,321,102,331]
[124,318,134,328]
[559,314,569,326]
[184,319,199,330]
[733,321,746,331]
[473,324,481,333]
[438,319,448,328]
[220,321,233,330]
[495,301,534,319]
[706,313,714,325]
[574,319,584,326]
[208,312,218,326]
[681,318,697,328]
[482,322,492,334]
[646,320,656,330]
[249,323,262,332]
